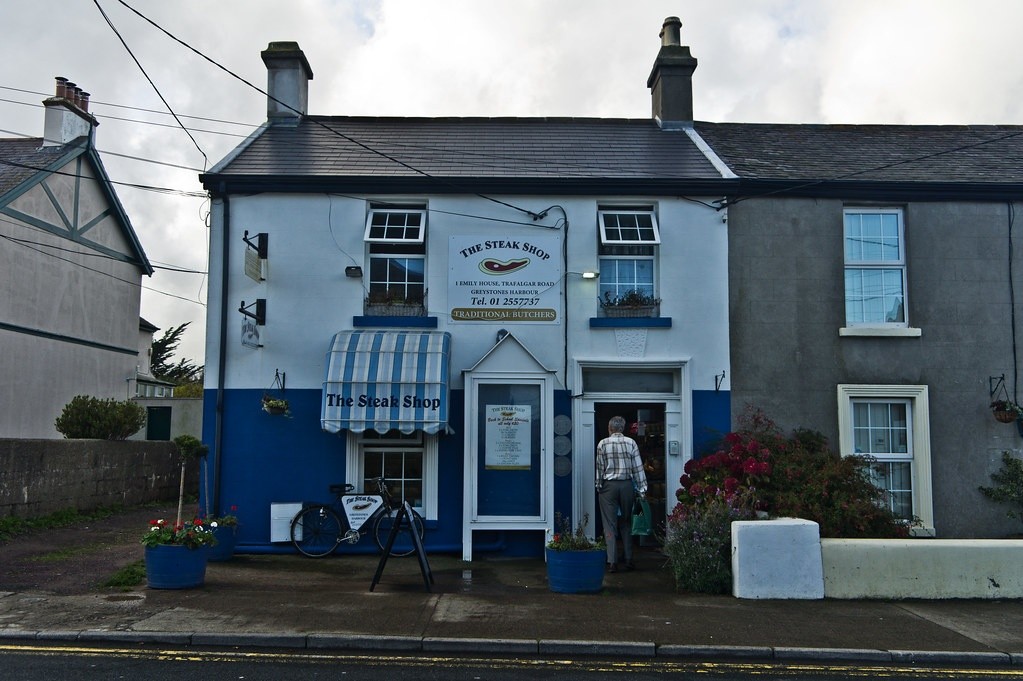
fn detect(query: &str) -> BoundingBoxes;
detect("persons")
[596,416,648,572]
[643,456,654,470]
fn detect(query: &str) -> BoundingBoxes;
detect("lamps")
[580,271,600,279]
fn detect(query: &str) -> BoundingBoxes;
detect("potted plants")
[365,292,424,316]
[262,394,289,416]
[596,288,662,317]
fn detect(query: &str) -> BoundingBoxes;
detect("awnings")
[321,329,450,434]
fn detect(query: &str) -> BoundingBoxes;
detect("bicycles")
[288,476,425,558]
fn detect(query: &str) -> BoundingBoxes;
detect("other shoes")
[624,559,633,567]
[608,563,616,572]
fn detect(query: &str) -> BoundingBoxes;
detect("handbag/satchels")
[632,497,651,536]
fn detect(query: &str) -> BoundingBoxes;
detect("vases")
[144,543,208,589]
[545,545,608,594]
[205,526,238,561]
[994,411,1018,423]
[701,576,729,596]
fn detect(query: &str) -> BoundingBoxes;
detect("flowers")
[676,396,937,539]
[138,517,220,549]
[546,511,606,550]
[192,506,237,526]
[990,400,1023,414]
[659,505,754,578]
